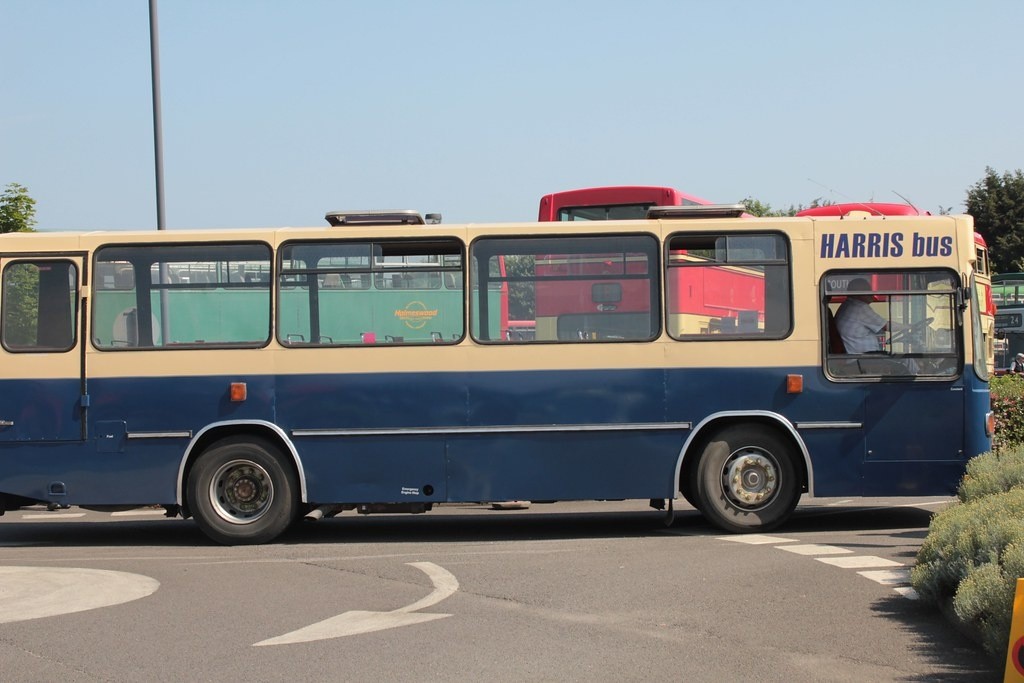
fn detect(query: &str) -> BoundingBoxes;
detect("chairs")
[119,267,456,289]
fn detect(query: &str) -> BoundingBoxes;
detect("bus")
[536,187,767,340]
[796,200,995,376]
[66,250,508,348]
[0,202,998,548]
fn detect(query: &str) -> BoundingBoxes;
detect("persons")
[834,278,920,354]
[1010,353,1024,378]
